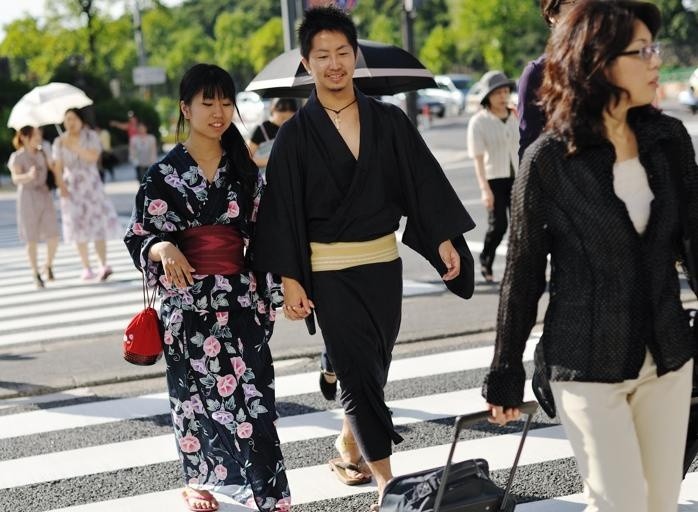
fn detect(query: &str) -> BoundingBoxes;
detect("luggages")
[381,402,539,511]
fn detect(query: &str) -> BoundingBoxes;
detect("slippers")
[330,458,372,485]
[182,491,217,511]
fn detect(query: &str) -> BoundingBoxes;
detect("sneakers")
[478,256,492,282]
[34,265,110,289]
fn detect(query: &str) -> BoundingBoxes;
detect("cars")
[383,74,472,116]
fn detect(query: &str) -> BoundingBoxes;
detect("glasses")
[619,41,661,59]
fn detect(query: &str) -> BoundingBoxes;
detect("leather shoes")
[320,364,337,400]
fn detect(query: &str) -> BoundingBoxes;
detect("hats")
[479,70,516,106]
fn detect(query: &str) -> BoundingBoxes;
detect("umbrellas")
[7,82,95,138]
[243,34,439,98]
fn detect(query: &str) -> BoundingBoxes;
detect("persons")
[87,108,161,186]
[122,64,316,511]
[465,69,528,283]
[46,106,123,281]
[249,93,297,186]
[244,7,475,510]
[482,2,698,510]
[518,0,577,420]
[318,344,337,402]
[7,125,68,287]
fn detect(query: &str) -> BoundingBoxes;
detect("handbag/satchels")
[122,308,163,366]
[47,170,57,191]
